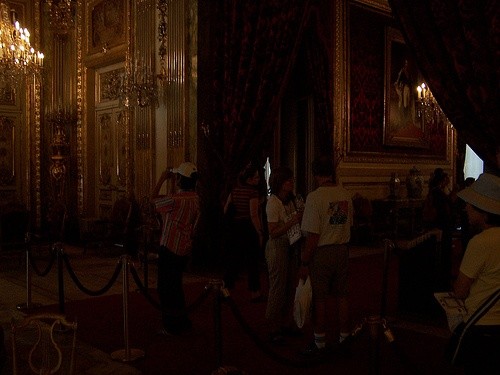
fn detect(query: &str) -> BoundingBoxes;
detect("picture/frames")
[382,27,425,148]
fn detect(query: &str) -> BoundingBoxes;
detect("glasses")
[254,176,261,179]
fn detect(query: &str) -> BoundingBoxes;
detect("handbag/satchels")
[293,275,313,332]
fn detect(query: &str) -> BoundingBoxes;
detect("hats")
[311,161,332,176]
[456,173,500,216]
[172,162,198,179]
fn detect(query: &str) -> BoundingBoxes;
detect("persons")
[295,159,354,360]
[150,163,201,335]
[225,168,263,304]
[265,166,304,347]
[451,173,500,375]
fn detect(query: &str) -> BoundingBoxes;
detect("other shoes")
[313,333,327,349]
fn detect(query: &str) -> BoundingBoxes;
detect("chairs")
[81,199,129,256]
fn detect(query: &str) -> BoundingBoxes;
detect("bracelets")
[301,262,310,266]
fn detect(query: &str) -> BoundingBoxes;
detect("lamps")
[120,0,175,110]
[416,83,448,123]
[0,0,44,91]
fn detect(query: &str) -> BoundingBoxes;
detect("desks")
[357,198,425,239]
